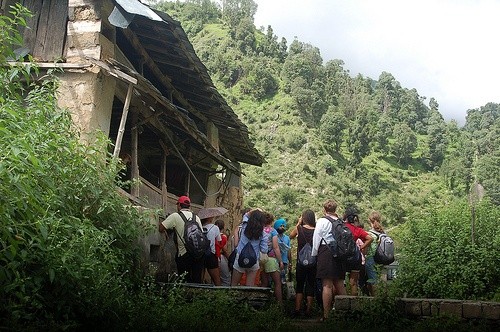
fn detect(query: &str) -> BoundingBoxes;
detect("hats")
[274,219,286,231]
[176,196,191,206]
[215,220,224,227]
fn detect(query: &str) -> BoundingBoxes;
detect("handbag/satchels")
[287,270,296,300]
[346,245,361,267]
[228,249,236,271]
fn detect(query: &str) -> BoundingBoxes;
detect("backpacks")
[201,226,215,256]
[369,230,394,264]
[321,216,355,263]
[239,240,257,268]
[299,226,316,268]
[178,211,209,259]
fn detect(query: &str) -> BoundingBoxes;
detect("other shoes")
[294,310,300,315]
[318,317,331,323]
[305,308,313,316]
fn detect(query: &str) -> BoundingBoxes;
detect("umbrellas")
[196,207,229,220]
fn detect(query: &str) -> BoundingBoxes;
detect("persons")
[158,196,203,284]
[341,209,386,297]
[200,220,224,286]
[290,209,319,316]
[231,208,289,317]
[311,200,347,323]
